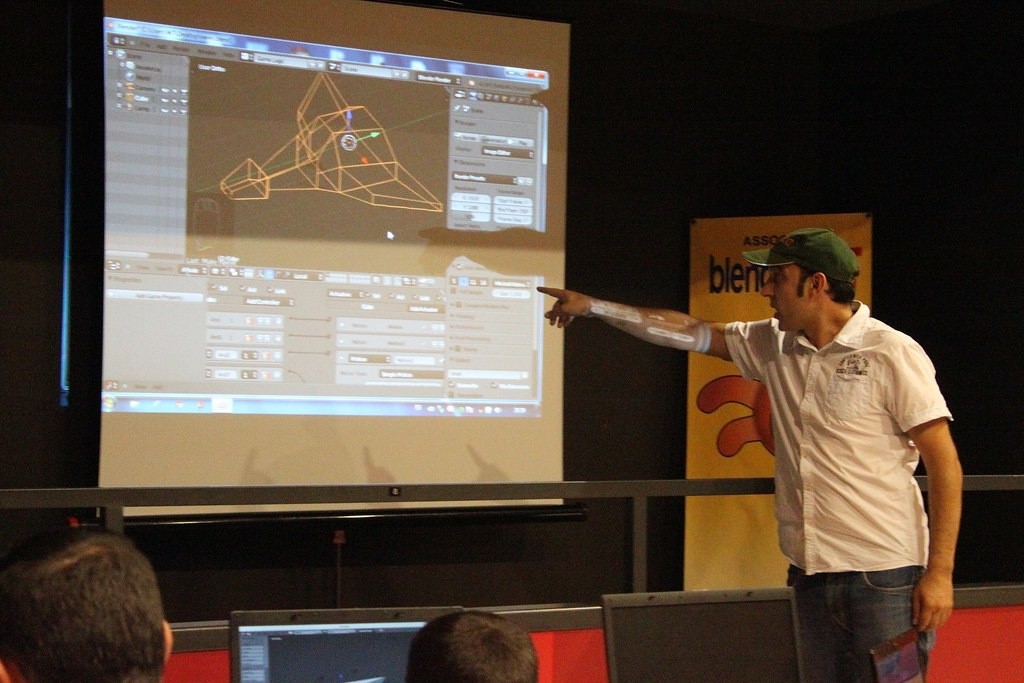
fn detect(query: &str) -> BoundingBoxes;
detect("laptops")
[603,586,805,683]
[232,606,465,683]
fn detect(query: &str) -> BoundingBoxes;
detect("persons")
[0,523,173,683]
[402,610,539,683]
[536,226,963,683]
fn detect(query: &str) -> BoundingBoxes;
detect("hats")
[741,228,861,285]
